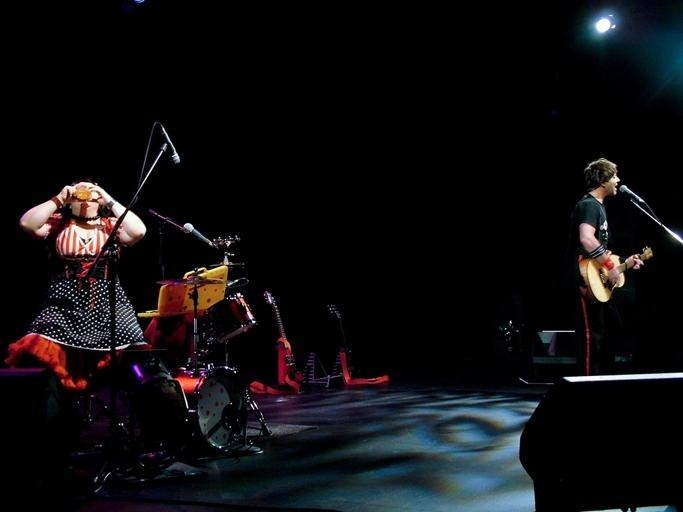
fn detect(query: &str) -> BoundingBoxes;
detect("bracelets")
[599,258,613,271]
[589,244,605,259]
[105,198,117,210]
[51,196,63,209]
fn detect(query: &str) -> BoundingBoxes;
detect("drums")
[175,366,248,454]
[210,293,257,343]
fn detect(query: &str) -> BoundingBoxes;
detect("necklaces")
[68,211,100,221]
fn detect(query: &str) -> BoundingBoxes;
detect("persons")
[565,155,645,376]
[3,181,148,433]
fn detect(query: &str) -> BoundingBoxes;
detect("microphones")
[183,222,218,251]
[158,125,181,165]
[619,184,645,204]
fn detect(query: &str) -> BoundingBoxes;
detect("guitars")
[326,304,354,385]
[579,245,654,302]
[263,290,305,394]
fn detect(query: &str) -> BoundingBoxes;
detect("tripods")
[222,340,273,435]
[78,153,172,493]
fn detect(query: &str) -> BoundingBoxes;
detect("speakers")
[518,372,683,512]
[0,368,47,512]
